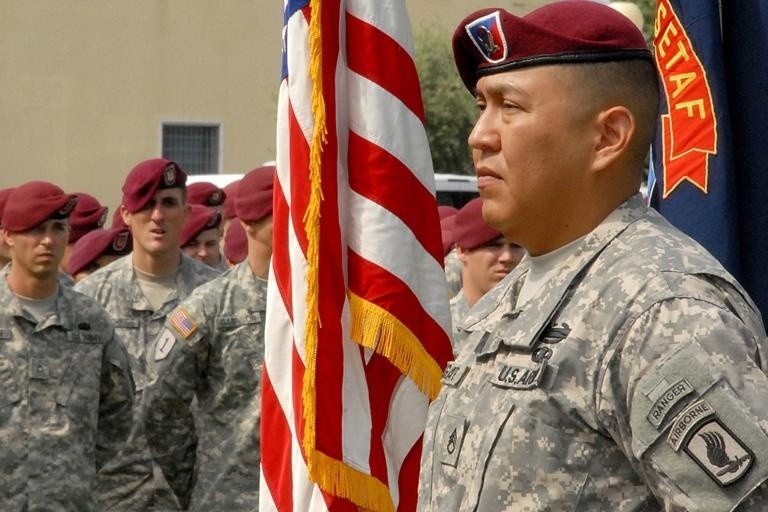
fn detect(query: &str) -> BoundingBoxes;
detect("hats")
[0,157,275,276]
[438,197,502,256]
[451,1,656,99]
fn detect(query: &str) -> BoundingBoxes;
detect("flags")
[256,0,453,511]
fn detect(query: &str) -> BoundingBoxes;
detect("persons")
[139,169,277,511]
[75,158,226,384]
[178,178,248,274]
[416,1,768,511]
[62,192,134,287]
[1,183,137,512]
[438,199,522,355]
[2,187,18,266]
